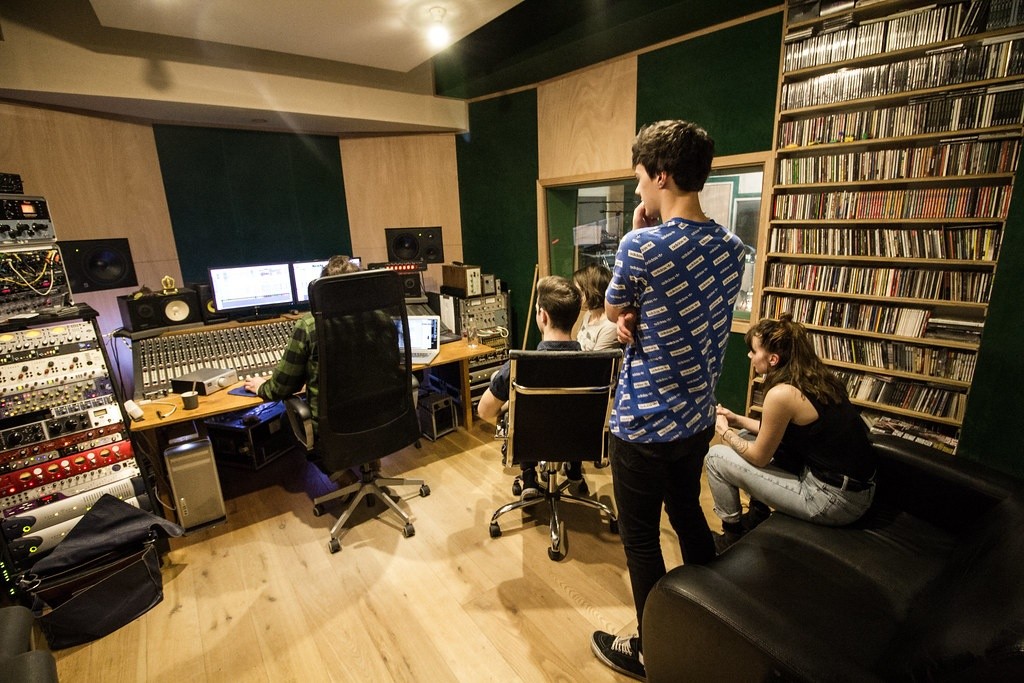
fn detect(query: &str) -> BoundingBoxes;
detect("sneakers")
[590,630,647,682]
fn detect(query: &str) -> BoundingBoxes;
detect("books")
[751,0,1024,458]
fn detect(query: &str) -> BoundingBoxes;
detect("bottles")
[467,313,478,348]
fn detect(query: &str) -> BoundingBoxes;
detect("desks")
[130,338,494,524]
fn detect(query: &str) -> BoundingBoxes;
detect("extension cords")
[124,400,142,419]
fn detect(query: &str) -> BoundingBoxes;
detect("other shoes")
[569,480,590,499]
[359,459,383,474]
[741,503,770,531]
[521,470,537,500]
[336,468,361,502]
[711,529,731,555]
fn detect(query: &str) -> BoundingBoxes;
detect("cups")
[180,390,199,410]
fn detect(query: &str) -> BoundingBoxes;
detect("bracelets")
[723,429,733,441]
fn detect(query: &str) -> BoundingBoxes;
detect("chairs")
[281,269,430,554]
[490,349,625,561]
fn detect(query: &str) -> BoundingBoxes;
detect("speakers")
[193,283,244,321]
[385,226,445,264]
[116,286,202,332]
[395,274,421,298]
[56,238,139,293]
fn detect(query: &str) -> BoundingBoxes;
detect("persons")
[573,262,618,352]
[245,253,399,508]
[476,275,590,497]
[703,313,877,555]
[592,121,745,683]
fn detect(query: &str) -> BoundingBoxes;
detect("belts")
[808,468,873,492]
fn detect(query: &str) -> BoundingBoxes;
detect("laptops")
[390,315,441,365]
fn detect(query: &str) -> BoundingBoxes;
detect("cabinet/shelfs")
[745,0,1024,453]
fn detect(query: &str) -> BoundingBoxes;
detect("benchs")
[644,432,1024,683]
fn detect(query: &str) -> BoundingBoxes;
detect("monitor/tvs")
[208,256,362,322]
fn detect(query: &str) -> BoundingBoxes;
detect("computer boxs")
[163,422,228,536]
